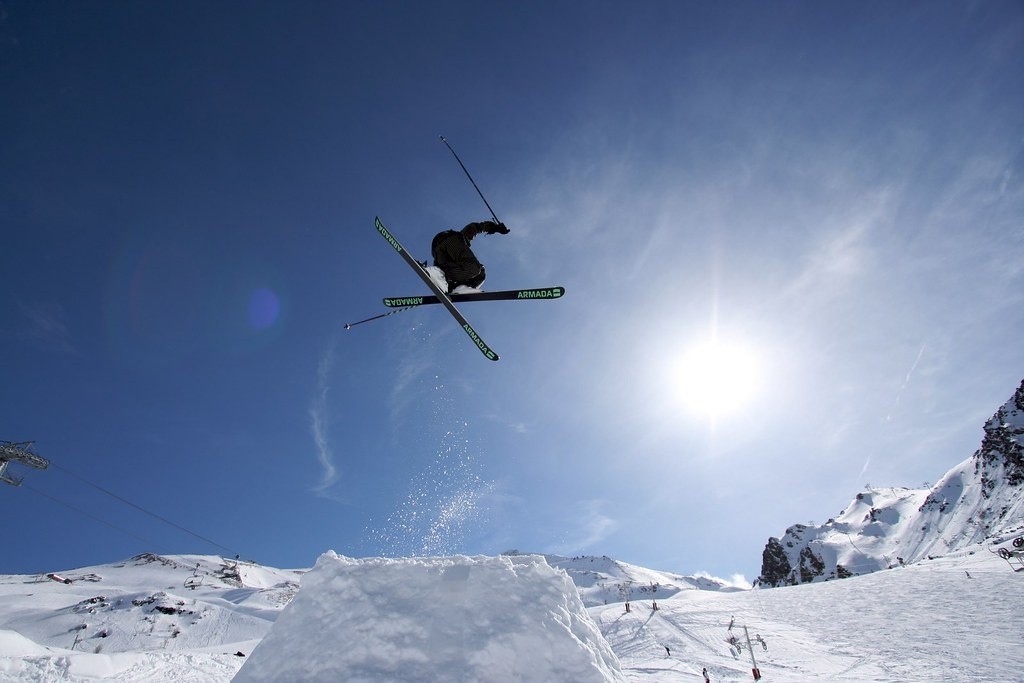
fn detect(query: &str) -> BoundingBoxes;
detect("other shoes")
[424,266,449,294]
[450,284,484,294]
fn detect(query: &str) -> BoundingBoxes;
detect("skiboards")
[374,213,565,361]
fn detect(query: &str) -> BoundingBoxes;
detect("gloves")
[496,223,510,234]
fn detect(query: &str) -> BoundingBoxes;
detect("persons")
[425,221,510,295]
[703,668,710,683]
